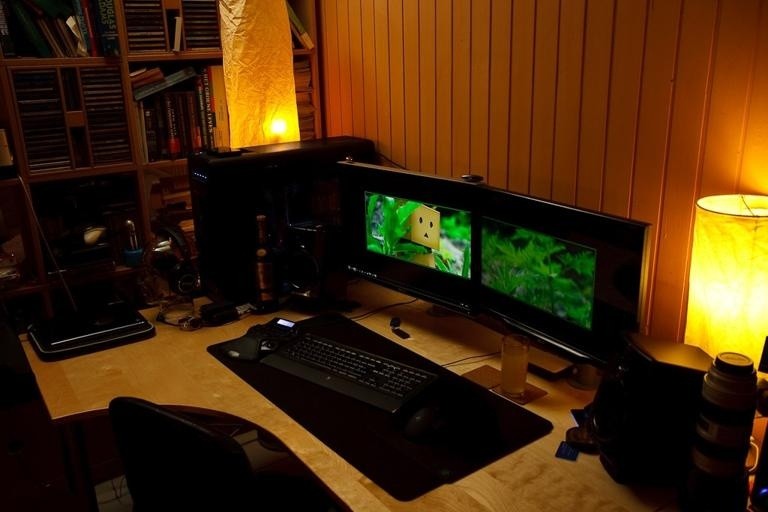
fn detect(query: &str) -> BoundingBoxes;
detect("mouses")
[405,406,448,435]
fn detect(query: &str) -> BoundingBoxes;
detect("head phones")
[154,222,198,296]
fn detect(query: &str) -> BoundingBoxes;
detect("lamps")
[684,192,767,374]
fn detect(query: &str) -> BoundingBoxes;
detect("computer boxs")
[187,136,377,307]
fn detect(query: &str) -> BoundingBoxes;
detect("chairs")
[107,393,341,512]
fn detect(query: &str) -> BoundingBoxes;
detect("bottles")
[693,349,766,483]
[252,213,284,314]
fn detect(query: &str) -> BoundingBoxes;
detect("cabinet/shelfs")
[0,0,326,325]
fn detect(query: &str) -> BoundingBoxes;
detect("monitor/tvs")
[337,158,480,316]
[480,183,649,371]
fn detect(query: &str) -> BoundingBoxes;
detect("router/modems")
[527,344,574,380]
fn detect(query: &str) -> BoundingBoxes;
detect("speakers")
[590,333,716,488]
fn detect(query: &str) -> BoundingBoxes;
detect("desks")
[18,275,671,511]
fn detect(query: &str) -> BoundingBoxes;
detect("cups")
[500,334,531,398]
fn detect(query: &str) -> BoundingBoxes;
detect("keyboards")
[258,332,439,415]
